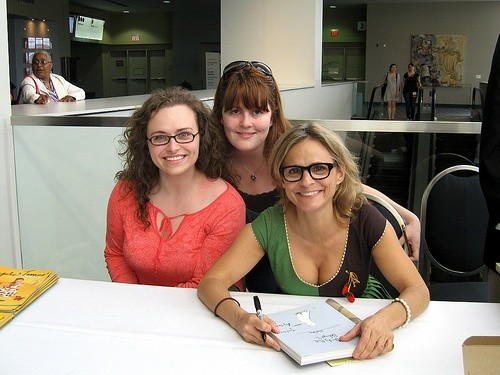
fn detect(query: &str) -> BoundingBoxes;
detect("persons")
[211,60,422,295]
[105,92,245,294]
[384,64,400,119]
[17,50,85,104]
[402,62,422,120]
[197,121,432,361]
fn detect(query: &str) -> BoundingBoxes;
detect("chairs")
[357,166,490,282]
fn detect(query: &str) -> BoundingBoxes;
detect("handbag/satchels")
[412,92,417,98]
[381,73,388,97]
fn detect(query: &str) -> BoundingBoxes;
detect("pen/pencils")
[253,296,267,343]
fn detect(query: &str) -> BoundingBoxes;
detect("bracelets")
[390,298,411,328]
[214,297,240,317]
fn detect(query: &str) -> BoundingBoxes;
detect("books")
[261,300,394,366]
[0,264,62,330]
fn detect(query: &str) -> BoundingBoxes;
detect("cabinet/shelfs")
[110,47,167,96]
[322,45,366,82]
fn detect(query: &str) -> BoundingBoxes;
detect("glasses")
[146,130,200,146]
[223,61,272,81]
[32,60,52,64]
[279,161,337,182]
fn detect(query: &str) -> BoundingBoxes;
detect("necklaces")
[237,155,267,180]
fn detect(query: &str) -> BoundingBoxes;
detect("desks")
[0,277,499,375]
